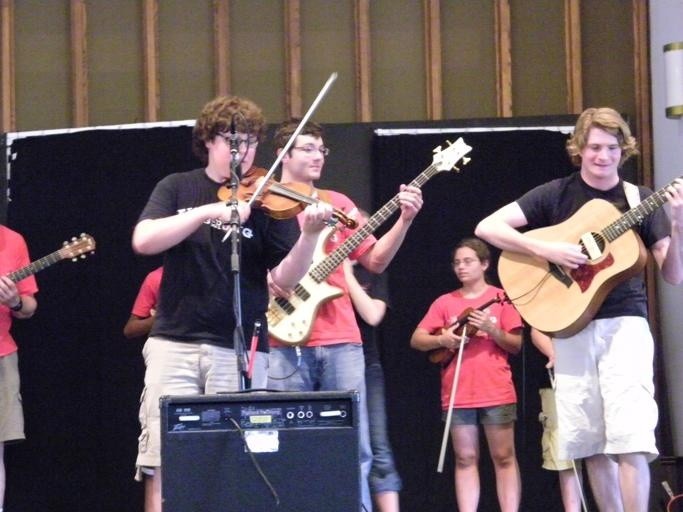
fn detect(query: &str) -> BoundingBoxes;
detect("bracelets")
[437,333,443,351]
[8,296,25,314]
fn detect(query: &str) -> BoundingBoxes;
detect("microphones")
[228,130,239,152]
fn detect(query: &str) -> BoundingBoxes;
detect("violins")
[425,292,512,363]
[218,167,358,231]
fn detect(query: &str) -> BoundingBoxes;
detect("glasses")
[217,132,258,148]
[294,145,329,156]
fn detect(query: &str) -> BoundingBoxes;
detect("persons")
[527,324,584,512]
[409,237,528,512]
[122,262,165,341]
[472,105,682,512]
[0,224,40,512]
[130,94,334,512]
[341,210,407,512]
[266,117,425,512]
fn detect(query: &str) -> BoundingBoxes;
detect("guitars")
[264,136,472,346]
[498,176,683,338]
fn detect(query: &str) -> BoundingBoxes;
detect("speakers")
[159,391,359,511]
